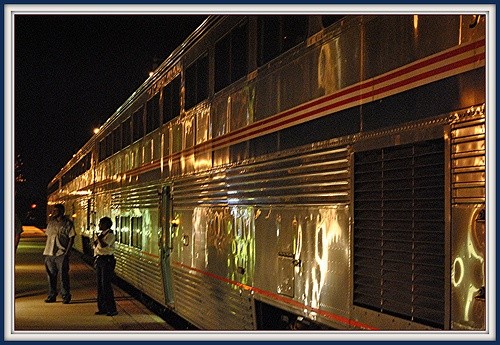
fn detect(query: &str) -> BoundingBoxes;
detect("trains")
[46,13,487,332]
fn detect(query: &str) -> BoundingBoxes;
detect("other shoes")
[95,311,106,315]
[62,298,70,304]
[45,296,56,303]
[106,312,118,317]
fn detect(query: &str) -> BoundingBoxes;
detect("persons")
[15,214,23,249]
[90,215,118,316]
[42,204,76,304]
